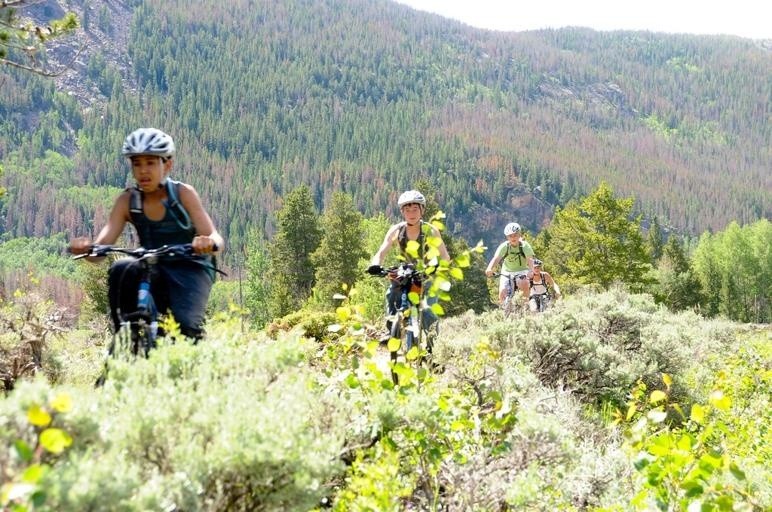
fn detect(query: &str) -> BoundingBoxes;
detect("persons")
[529,259,561,311]
[69,127,224,355]
[367,190,450,382]
[485,222,536,312]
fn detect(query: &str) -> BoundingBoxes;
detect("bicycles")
[66,244,226,369]
[487,268,537,323]
[526,290,562,313]
[365,261,447,391]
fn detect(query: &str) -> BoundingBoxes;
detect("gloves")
[365,265,383,275]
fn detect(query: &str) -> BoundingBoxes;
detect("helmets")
[398,191,426,208]
[502,223,521,235]
[122,128,178,157]
[533,259,543,266]
